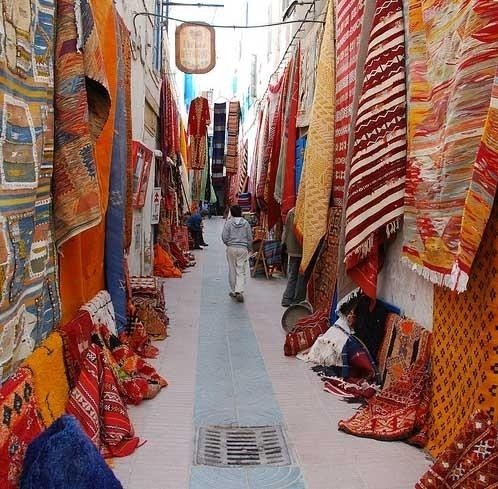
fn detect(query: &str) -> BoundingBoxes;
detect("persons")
[186,206,209,250]
[220,205,250,303]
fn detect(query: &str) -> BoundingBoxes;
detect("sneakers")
[229,291,243,301]
[193,243,208,249]
